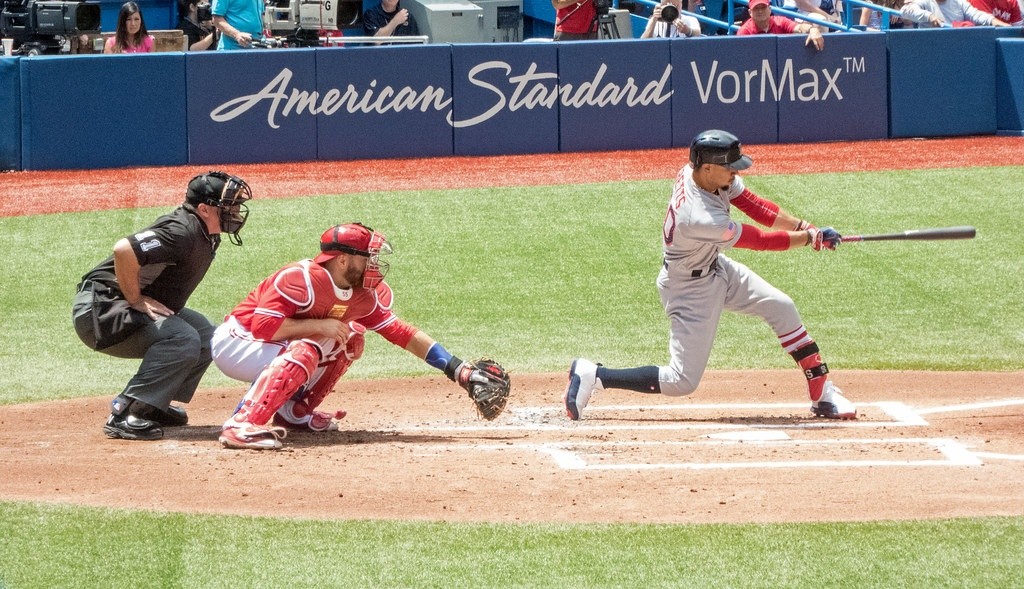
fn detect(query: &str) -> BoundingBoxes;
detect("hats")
[186,173,248,206]
[749,0,770,10]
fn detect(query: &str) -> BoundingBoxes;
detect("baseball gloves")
[458,356,511,422]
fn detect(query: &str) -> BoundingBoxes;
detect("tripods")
[587,14,620,40]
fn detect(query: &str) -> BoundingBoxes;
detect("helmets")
[689,131,753,169]
[316,223,384,264]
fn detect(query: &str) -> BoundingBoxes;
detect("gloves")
[794,221,843,251]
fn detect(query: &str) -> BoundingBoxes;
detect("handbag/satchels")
[91,280,149,351]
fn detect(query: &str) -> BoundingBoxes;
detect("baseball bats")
[839,224,979,243]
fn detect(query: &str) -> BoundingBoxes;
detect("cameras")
[658,4,680,22]
[197,3,214,21]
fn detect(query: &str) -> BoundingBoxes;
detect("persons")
[102,0,1024,67]
[72,170,254,442]
[211,222,511,448]
[566,130,857,420]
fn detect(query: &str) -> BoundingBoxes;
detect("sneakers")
[218,424,282,451]
[139,404,188,427]
[273,404,338,431]
[564,357,604,419]
[103,408,164,441]
[810,395,857,418]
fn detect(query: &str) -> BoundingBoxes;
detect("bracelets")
[689,29,693,37]
[807,26,817,33]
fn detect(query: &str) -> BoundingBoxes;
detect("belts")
[664,260,718,277]
[78,281,93,291]
